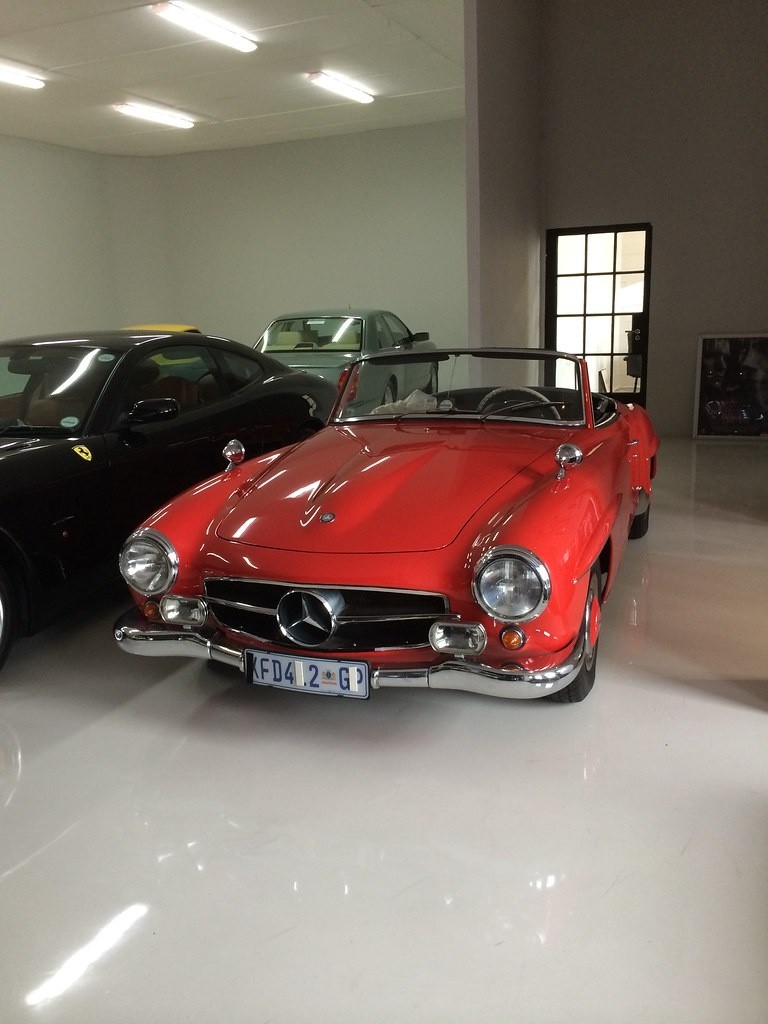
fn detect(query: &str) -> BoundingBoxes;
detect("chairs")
[268,330,318,349]
[326,329,361,350]
[28,398,57,424]
[146,372,198,414]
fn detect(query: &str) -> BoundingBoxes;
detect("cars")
[122,325,203,364]
[0,334,339,669]
[255,310,439,419]
[112,344,661,704]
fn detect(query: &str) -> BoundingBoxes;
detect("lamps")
[150,2,259,52]
[307,72,374,104]
[1,67,45,89]
[112,105,194,129]
